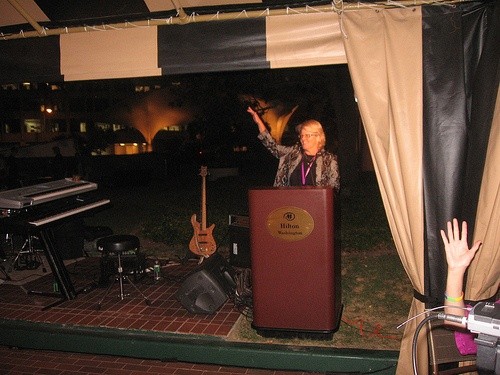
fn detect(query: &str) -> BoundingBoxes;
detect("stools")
[93,235,153,309]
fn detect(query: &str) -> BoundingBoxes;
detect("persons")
[441,218,500,355]
[247,104,341,195]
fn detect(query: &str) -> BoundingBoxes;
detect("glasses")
[297,133,319,139]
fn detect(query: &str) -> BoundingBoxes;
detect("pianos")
[0,176,112,301]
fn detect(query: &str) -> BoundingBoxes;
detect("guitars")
[189,165,217,256]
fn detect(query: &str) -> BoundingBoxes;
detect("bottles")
[154,261,161,281]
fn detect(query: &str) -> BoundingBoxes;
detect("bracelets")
[444,290,464,303]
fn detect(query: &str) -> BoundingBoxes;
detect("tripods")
[1,234,46,273]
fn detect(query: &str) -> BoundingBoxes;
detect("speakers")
[173,252,236,314]
[229,228,251,269]
[100,254,146,282]
[53,215,84,260]
